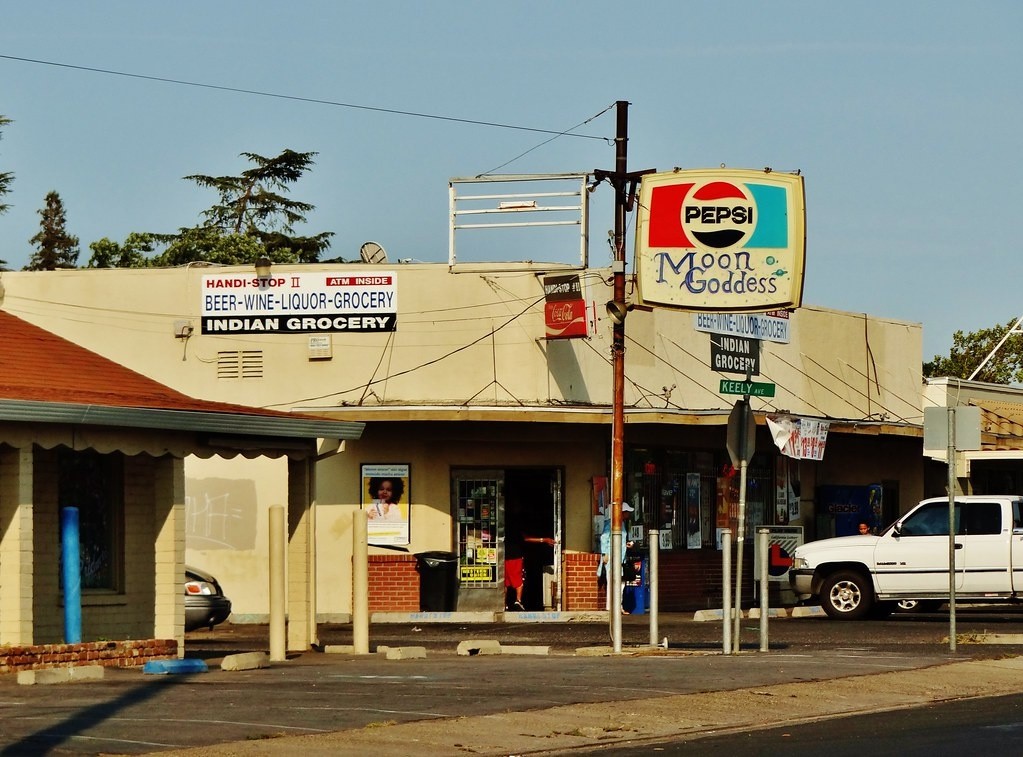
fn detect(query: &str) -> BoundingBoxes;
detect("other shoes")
[514,601,525,611]
[505,605,508,610]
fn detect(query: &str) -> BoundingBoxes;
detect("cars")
[184,565,234,632]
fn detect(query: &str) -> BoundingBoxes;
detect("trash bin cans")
[413,550,458,613]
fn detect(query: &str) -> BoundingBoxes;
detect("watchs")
[540,538,544,542]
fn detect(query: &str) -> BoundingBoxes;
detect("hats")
[621,502,635,512]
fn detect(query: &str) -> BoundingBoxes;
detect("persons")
[504,530,556,610]
[858,522,872,536]
[366,477,409,545]
[597,502,635,615]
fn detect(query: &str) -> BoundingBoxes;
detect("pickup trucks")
[786,490,1023,622]
